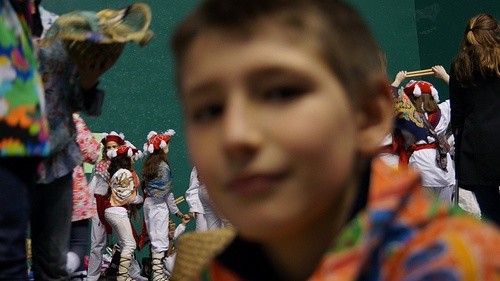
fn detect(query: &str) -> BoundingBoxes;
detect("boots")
[117,256,138,281]
[152,257,173,281]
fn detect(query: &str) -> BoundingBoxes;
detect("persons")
[169,0,500,281]
[0,0,99,281]
[89,113,233,281]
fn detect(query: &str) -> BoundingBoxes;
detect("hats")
[404,80,436,95]
[143,129,176,154]
[101,131,126,146]
[109,146,139,158]
